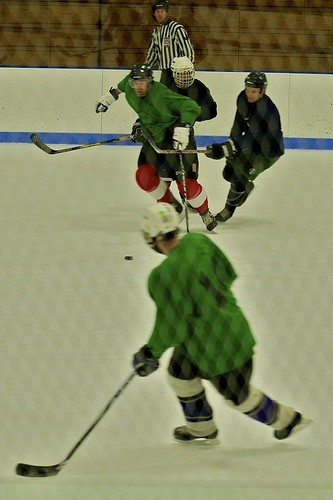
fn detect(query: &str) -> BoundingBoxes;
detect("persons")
[130,56,217,212]
[133,202,314,444]
[206,72,283,223]
[143,0,195,89]
[94,61,217,232]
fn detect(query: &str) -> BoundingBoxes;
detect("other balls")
[124,255,133,261]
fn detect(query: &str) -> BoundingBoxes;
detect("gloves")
[205,138,242,159]
[172,123,191,151]
[133,345,160,377]
[131,118,146,143]
[95,86,118,113]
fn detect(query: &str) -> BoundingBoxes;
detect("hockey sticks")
[180,150,189,233]
[16,369,138,478]
[32,132,133,154]
[143,135,212,155]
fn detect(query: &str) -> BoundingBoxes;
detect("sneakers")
[274,412,313,440]
[237,182,254,207]
[215,208,233,224]
[174,426,221,445]
[170,200,185,224]
[199,208,221,234]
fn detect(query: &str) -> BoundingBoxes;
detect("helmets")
[171,56,195,89]
[245,72,268,89]
[130,64,152,80]
[140,202,177,237]
[151,0,169,9]
